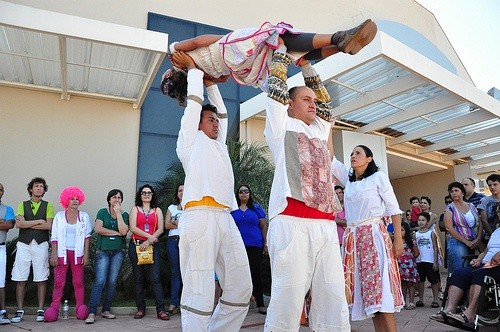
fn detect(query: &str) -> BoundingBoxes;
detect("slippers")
[448,312,478,330]
[416,300,423,306]
[430,311,446,322]
[430,302,439,307]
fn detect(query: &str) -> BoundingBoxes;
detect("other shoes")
[85,314,96,323]
[101,312,115,318]
[259,310,267,315]
[407,303,415,309]
[134,310,144,318]
[157,311,170,320]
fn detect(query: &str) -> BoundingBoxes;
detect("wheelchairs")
[430,254,500,332]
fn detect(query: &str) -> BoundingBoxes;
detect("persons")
[172,50,252,332]
[10,177,54,322]
[444,182,483,274]
[231,186,267,314]
[85,189,129,323]
[0,183,15,323]
[129,183,170,320]
[464,178,485,207]
[326,117,405,332]
[335,185,347,252]
[45,187,91,322]
[387,196,445,309]
[477,174,500,242]
[429,202,500,322]
[264,37,351,332]
[165,184,182,314]
[160,19,377,87]
[439,195,453,268]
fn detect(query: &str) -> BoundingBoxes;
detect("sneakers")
[0,310,10,324]
[11,310,24,322]
[36,310,45,321]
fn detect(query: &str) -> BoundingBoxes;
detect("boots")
[333,19,371,53]
[350,22,377,55]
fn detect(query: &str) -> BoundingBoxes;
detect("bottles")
[62,300,69,319]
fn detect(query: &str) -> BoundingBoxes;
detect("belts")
[170,236,179,239]
[0,242,5,245]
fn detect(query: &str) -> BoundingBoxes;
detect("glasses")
[445,201,452,205]
[238,189,250,194]
[406,214,411,216]
[141,192,154,195]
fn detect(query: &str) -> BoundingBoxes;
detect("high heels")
[166,306,178,315]
